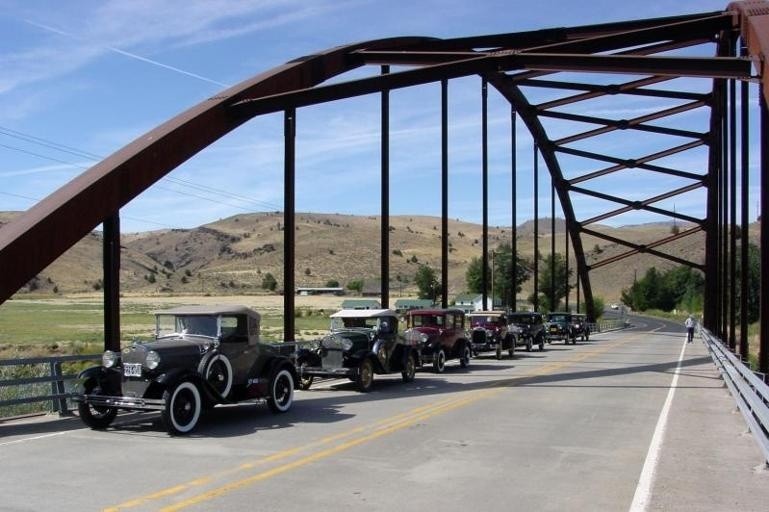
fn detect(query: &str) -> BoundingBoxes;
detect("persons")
[684,313,697,343]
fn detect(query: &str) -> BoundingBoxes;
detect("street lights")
[490,249,506,309]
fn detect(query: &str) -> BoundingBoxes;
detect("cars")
[610,304,620,310]
